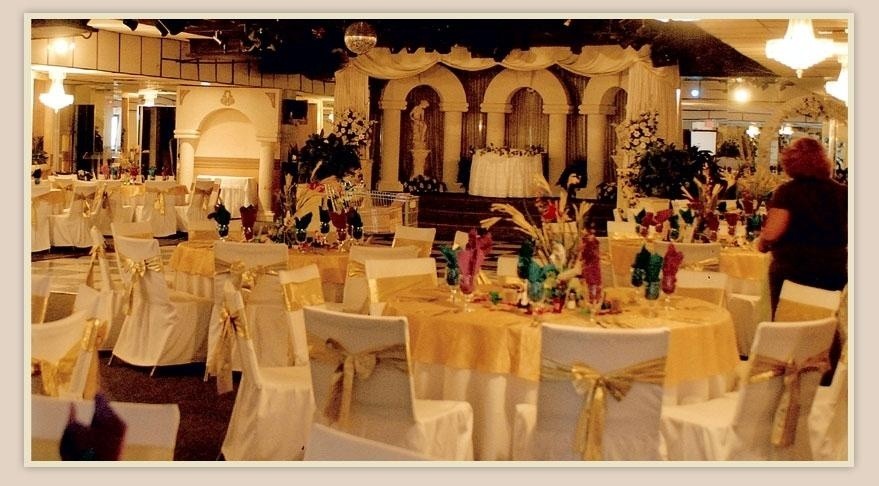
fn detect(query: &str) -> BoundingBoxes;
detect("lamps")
[41,71,73,112]
[823,63,850,109]
[345,20,379,54]
[762,14,837,77]
[140,87,159,106]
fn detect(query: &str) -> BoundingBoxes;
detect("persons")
[410,100,429,142]
[755,136,848,324]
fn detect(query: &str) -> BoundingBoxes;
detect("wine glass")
[444,267,678,324]
[101,164,169,185]
[633,220,765,244]
[213,221,365,255]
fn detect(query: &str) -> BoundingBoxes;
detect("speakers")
[283,99,308,125]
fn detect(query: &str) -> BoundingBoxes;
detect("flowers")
[614,107,663,151]
[465,142,545,157]
[794,95,831,121]
[335,107,379,147]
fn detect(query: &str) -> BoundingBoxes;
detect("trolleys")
[324,180,422,246]
